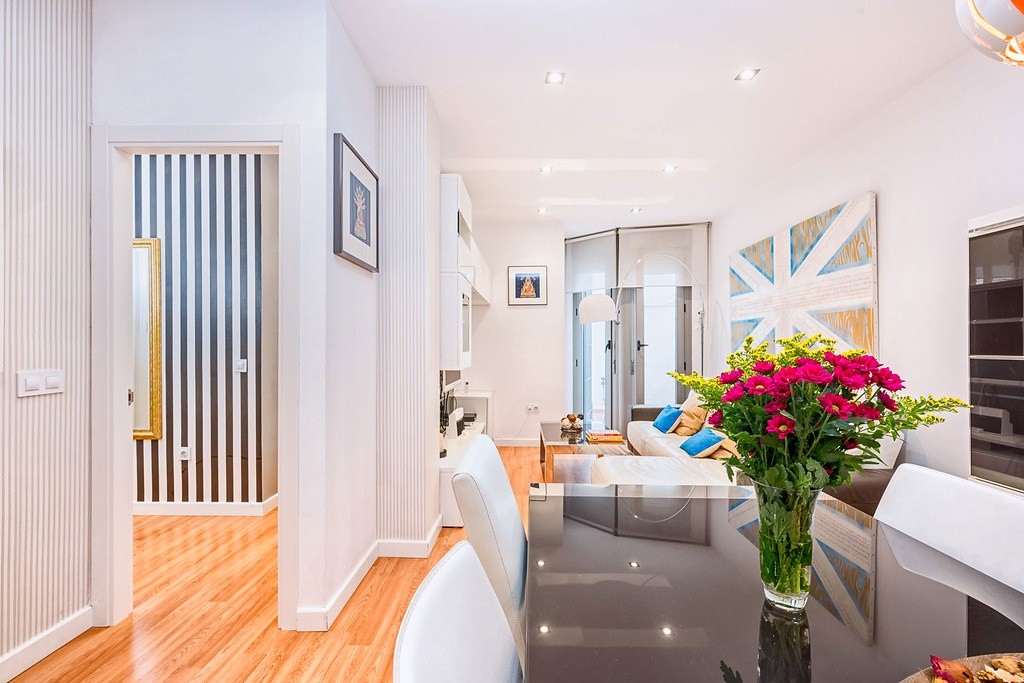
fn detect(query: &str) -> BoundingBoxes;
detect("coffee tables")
[538,422,635,482]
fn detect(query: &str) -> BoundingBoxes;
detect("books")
[585,430,622,441]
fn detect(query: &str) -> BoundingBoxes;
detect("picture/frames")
[132,238,163,440]
[508,265,547,305]
[334,133,380,274]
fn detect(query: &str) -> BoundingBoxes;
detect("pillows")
[679,428,725,457]
[710,437,748,461]
[673,399,708,436]
[652,404,684,434]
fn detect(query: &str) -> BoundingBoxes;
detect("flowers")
[667,331,974,683]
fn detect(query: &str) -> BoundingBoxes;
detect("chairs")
[452,434,527,673]
[393,541,526,683]
[873,463,1024,591]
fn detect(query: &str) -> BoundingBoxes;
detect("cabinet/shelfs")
[440,172,491,370]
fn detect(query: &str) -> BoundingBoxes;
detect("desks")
[454,389,492,438]
[529,484,1023,683]
[438,424,486,528]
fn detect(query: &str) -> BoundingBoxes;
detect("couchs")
[625,400,905,515]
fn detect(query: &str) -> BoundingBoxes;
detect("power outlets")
[526,403,540,414]
[178,446,190,460]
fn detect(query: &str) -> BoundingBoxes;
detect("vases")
[746,474,824,683]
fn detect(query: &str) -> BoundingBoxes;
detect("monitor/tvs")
[443,370,462,392]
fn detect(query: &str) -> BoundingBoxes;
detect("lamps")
[955,0,1024,67]
[580,294,620,429]
